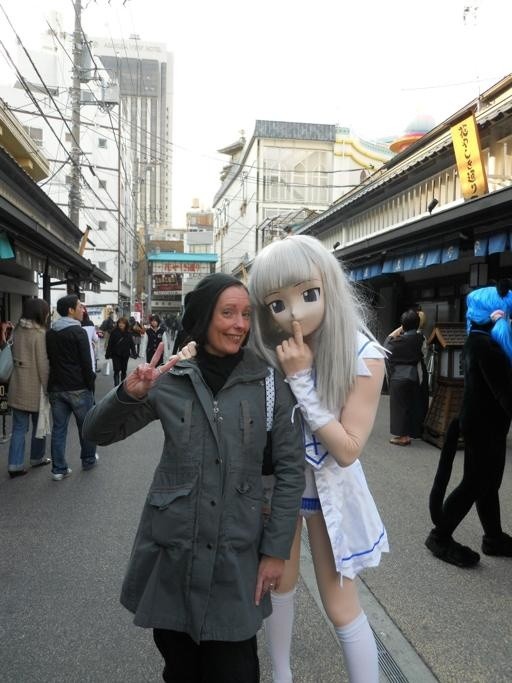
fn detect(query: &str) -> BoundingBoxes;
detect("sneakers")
[30,456,51,466]
[7,462,28,476]
[51,468,74,480]
[388,433,415,446]
[82,452,102,472]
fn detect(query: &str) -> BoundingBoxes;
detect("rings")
[269,583,275,587]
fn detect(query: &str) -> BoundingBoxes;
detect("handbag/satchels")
[101,358,113,378]
[0,343,14,382]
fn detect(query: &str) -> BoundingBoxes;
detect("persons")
[246,233,390,682]
[381,309,427,446]
[1,294,184,481]
[82,273,307,683]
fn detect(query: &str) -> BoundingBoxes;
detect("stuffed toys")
[425,284,511,567]
